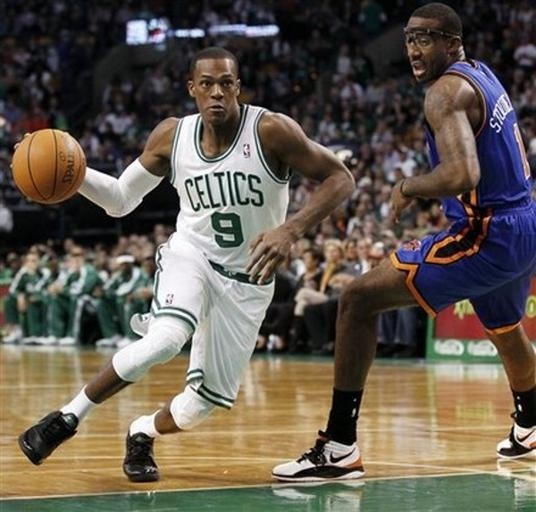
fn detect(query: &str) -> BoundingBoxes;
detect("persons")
[265,3,536,483]
[10,45,358,482]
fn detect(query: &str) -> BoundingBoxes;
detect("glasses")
[402,31,438,52]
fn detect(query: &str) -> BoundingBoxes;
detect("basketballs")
[12,129,86,205]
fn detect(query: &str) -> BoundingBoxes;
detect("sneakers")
[496,458,536,503]
[123,429,158,481]
[18,409,79,466]
[496,411,536,458]
[271,429,366,481]
[1,325,133,349]
[271,481,366,512]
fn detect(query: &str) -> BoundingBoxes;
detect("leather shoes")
[253,338,420,359]
